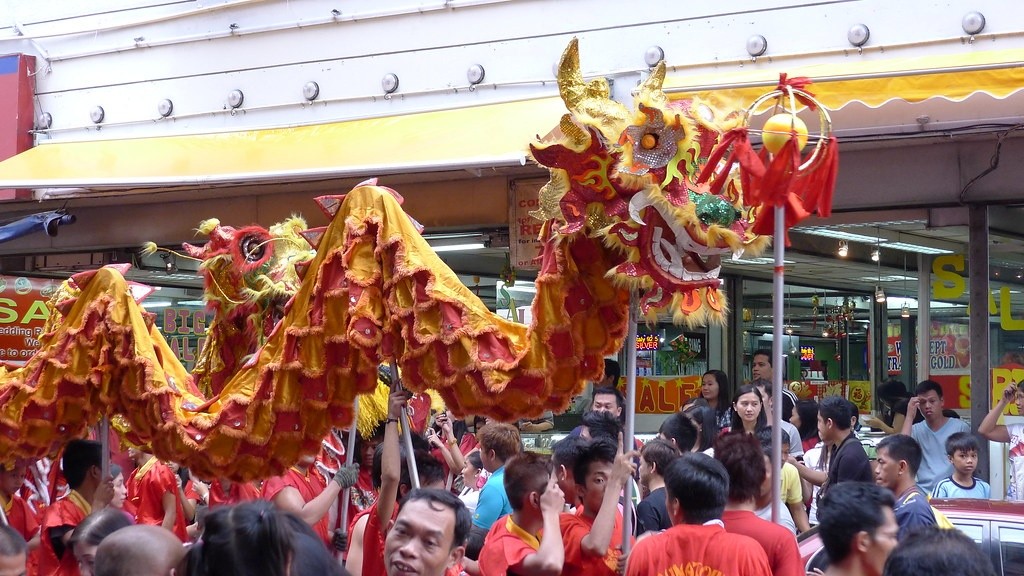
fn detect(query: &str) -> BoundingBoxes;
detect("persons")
[807,480,902,576]
[0,524,28,576]
[883,528,999,576]
[69,507,134,576]
[260,443,361,554]
[480,451,566,576]
[901,377,974,494]
[788,395,876,523]
[808,434,939,576]
[196,502,353,576]
[383,487,473,576]
[978,380,1024,502]
[94,522,187,576]
[559,430,642,576]
[928,430,994,501]
[709,431,806,576]
[0,352,959,575]
[622,450,773,576]
[38,439,115,576]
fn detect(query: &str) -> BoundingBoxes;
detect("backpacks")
[903,491,954,530]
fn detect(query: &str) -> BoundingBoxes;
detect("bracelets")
[447,438,458,446]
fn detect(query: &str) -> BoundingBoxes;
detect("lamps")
[788,335,798,354]
[39,112,51,129]
[900,251,910,319]
[158,98,173,117]
[962,10,986,35]
[874,224,884,303]
[871,246,882,262]
[230,22,241,34]
[785,285,793,335]
[838,238,849,257]
[848,22,870,46]
[466,63,485,92]
[643,45,664,66]
[161,251,179,276]
[228,89,243,116]
[89,105,104,123]
[331,8,343,19]
[381,73,398,99]
[134,36,144,46]
[303,81,319,105]
[746,35,768,56]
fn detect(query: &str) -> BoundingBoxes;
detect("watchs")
[385,418,400,424]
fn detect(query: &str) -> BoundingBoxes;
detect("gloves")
[331,528,348,551]
[333,461,360,488]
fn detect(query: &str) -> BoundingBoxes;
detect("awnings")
[0,97,569,188]
[631,44,1024,110]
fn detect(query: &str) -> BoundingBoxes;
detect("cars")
[797,498,1024,576]
